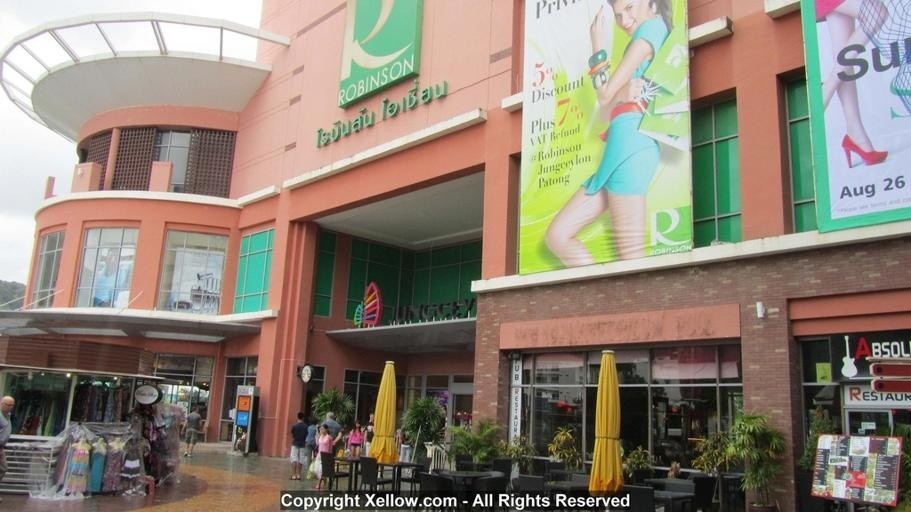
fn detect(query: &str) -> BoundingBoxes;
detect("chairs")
[358,456,393,497]
[401,456,432,497]
[318,450,348,497]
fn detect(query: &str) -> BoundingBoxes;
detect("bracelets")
[586,47,611,89]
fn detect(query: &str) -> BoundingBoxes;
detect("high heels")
[842,135,887,168]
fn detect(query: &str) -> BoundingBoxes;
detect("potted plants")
[690,414,784,512]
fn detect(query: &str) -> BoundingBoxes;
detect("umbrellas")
[585,349,628,511]
[369,360,401,478]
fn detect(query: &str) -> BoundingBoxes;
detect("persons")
[536,0,673,270]
[179,406,205,458]
[0,395,15,493]
[812,0,890,168]
[94,248,130,308]
[287,407,377,488]
